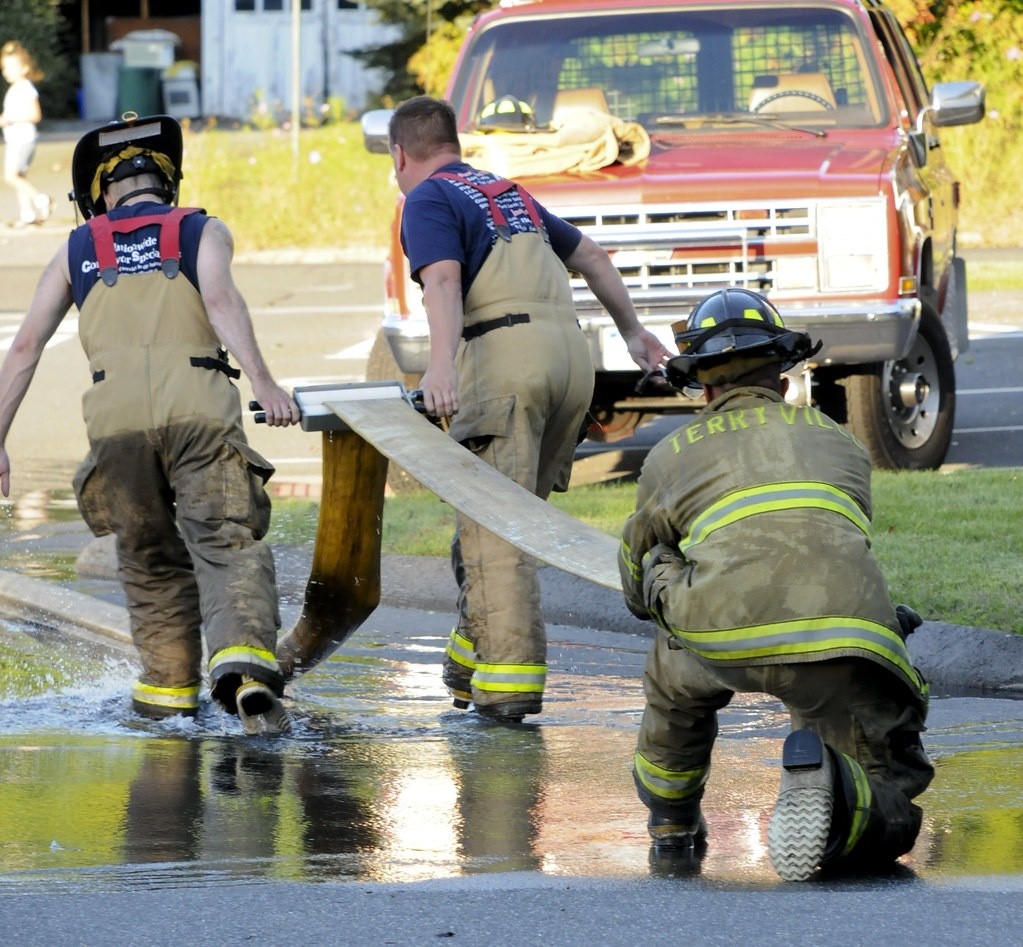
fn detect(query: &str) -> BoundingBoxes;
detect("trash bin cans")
[120,67,160,118]
[122,39,176,68]
[76,87,85,120]
[79,54,122,122]
[160,60,202,122]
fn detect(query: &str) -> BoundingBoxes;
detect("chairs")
[526,88,611,127]
[747,71,837,111]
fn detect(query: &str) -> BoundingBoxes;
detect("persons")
[388,96,677,723]
[619,288,934,880]
[0,111,300,734]
[0,42,55,227]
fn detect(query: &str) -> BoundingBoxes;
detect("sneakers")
[233,680,292,735]
[765,727,841,883]
[131,695,200,719]
[644,807,708,847]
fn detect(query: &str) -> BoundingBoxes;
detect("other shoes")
[473,699,544,723]
[442,682,475,711]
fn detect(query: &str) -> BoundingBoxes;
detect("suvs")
[361,0,987,474]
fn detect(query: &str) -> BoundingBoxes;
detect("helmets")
[666,287,830,389]
[70,114,186,220]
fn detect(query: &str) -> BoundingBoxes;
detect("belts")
[91,356,240,384]
[460,312,530,342]
